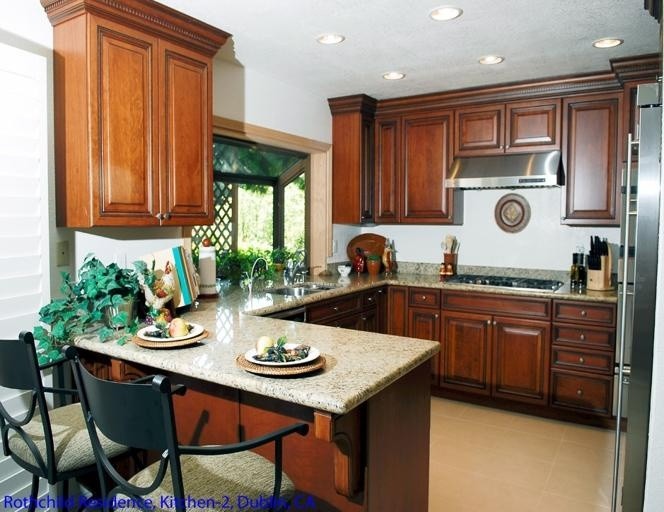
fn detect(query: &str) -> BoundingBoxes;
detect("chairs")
[66,347,309,512]
[0,330,151,512]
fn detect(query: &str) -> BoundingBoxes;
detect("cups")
[444,253,457,275]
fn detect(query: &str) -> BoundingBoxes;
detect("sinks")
[265,282,342,298]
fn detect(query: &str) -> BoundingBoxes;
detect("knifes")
[587,234,607,269]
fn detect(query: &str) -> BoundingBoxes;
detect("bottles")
[570,282,585,294]
[352,236,392,275]
[440,263,454,276]
[571,253,586,282]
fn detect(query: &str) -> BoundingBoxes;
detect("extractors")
[443,149,561,190]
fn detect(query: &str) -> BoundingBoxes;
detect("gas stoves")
[455,274,564,291]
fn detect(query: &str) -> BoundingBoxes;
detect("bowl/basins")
[337,265,352,276]
[338,276,351,284]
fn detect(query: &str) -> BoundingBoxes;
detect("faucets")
[247,258,268,298]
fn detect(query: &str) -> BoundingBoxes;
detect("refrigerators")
[618,83,664,512]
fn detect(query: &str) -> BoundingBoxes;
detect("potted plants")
[271,249,285,271]
[32,252,161,367]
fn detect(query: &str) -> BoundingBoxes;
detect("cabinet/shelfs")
[560,79,658,229]
[549,298,617,430]
[386,284,440,398]
[455,97,562,158]
[40,0,232,228]
[327,107,454,226]
[440,289,551,420]
[305,285,386,335]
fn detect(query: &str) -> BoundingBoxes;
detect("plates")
[244,342,320,366]
[137,320,205,342]
